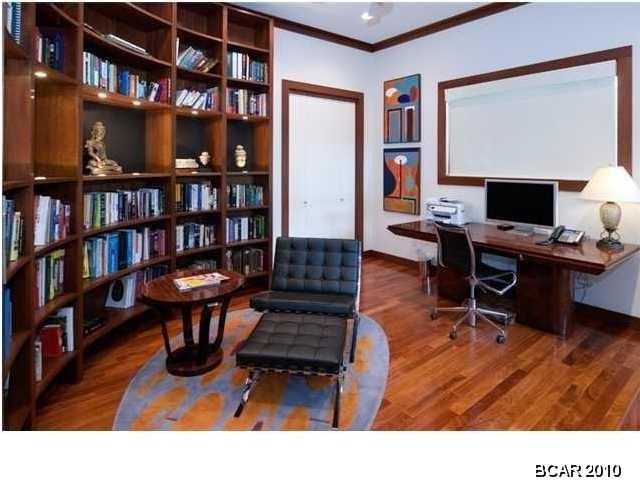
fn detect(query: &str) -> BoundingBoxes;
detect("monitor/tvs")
[483,179,558,236]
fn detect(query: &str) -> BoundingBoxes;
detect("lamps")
[574,162,639,251]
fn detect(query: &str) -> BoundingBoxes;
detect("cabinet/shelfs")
[2,2,275,431]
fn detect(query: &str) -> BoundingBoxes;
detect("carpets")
[110,306,393,431]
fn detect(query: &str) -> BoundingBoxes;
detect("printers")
[423,198,474,226]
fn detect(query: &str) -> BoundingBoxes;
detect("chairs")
[429,219,520,344]
[249,234,362,364]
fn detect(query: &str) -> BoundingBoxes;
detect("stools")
[233,310,348,429]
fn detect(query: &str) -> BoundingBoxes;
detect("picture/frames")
[382,72,422,145]
[382,147,422,217]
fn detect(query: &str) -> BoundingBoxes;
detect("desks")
[133,267,249,378]
[385,217,639,343]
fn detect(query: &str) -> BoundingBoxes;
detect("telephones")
[551,225,585,245]
